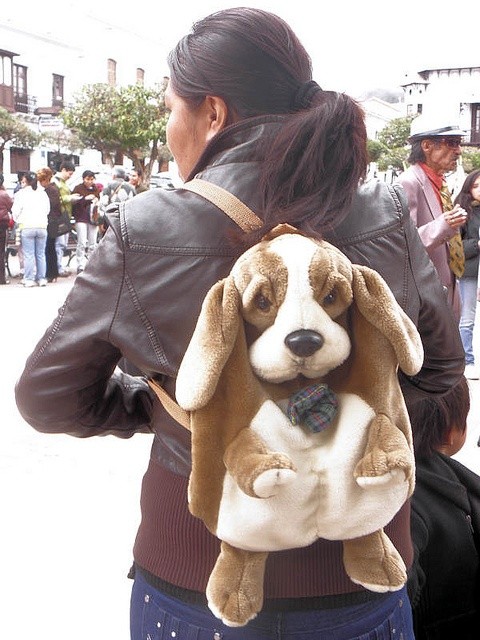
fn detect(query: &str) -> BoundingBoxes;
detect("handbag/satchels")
[46,209,72,239]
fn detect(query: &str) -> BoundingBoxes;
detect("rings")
[452,219,455,223]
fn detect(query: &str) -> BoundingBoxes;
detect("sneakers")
[20,275,35,287]
[36,278,47,287]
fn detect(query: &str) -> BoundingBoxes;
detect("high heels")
[46,274,59,283]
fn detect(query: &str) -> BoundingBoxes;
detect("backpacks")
[145,180,426,627]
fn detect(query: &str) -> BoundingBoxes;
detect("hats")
[408,112,468,140]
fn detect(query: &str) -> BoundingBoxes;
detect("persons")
[13,4,469,637]
[9,171,51,288]
[397,372,479,639]
[11,171,31,274]
[0,171,14,287]
[97,164,137,239]
[452,167,480,379]
[36,167,63,284]
[51,159,84,277]
[387,112,469,326]
[128,168,151,194]
[70,170,100,274]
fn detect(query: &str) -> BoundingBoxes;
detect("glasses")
[433,138,462,149]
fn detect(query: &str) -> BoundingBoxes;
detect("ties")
[438,179,465,281]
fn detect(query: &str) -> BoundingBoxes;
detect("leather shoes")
[58,271,69,278]
